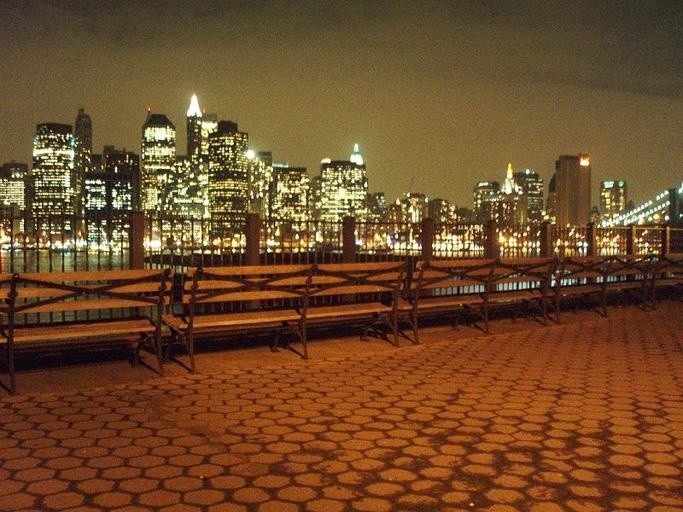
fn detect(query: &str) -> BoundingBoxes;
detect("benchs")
[157,256,415,377]
[553,249,669,326]
[0,265,179,399]
[653,251,683,310]
[407,252,561,344]
